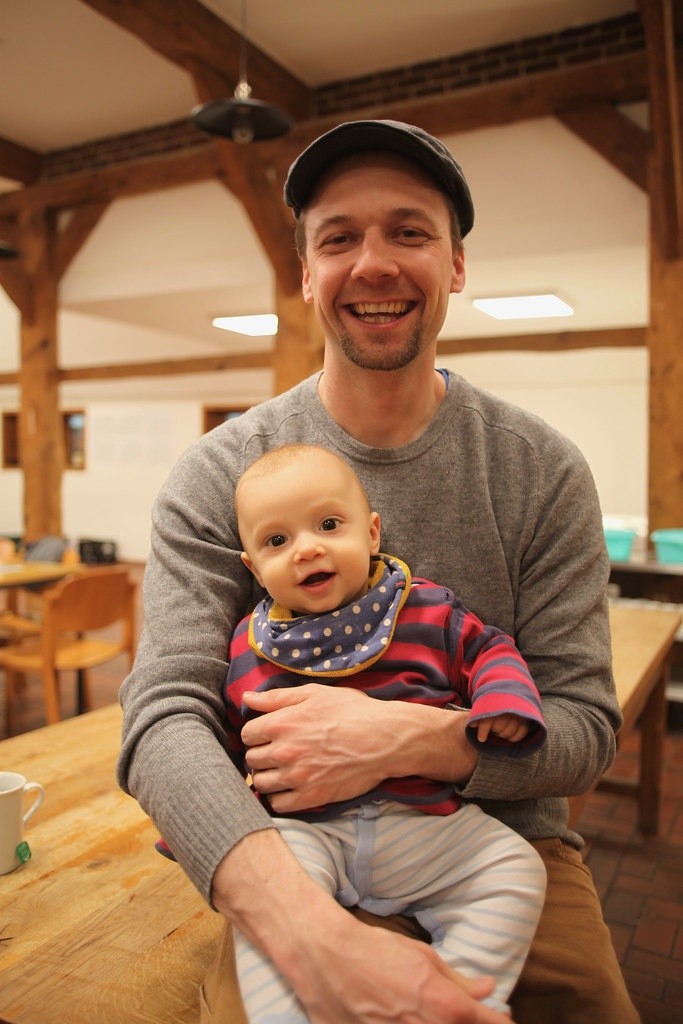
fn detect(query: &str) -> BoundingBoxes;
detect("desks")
[0,559,148,589]
[0,587,683,1024]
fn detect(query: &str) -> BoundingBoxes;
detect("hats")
[283,118,475,240]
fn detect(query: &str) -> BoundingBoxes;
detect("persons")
[153,445,549,1024]
[115,119,643,1024]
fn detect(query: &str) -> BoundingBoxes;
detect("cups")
[0,770,44,877]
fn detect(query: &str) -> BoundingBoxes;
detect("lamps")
[195,1,291,145]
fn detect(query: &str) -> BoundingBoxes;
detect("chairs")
[0,571,139,730]
